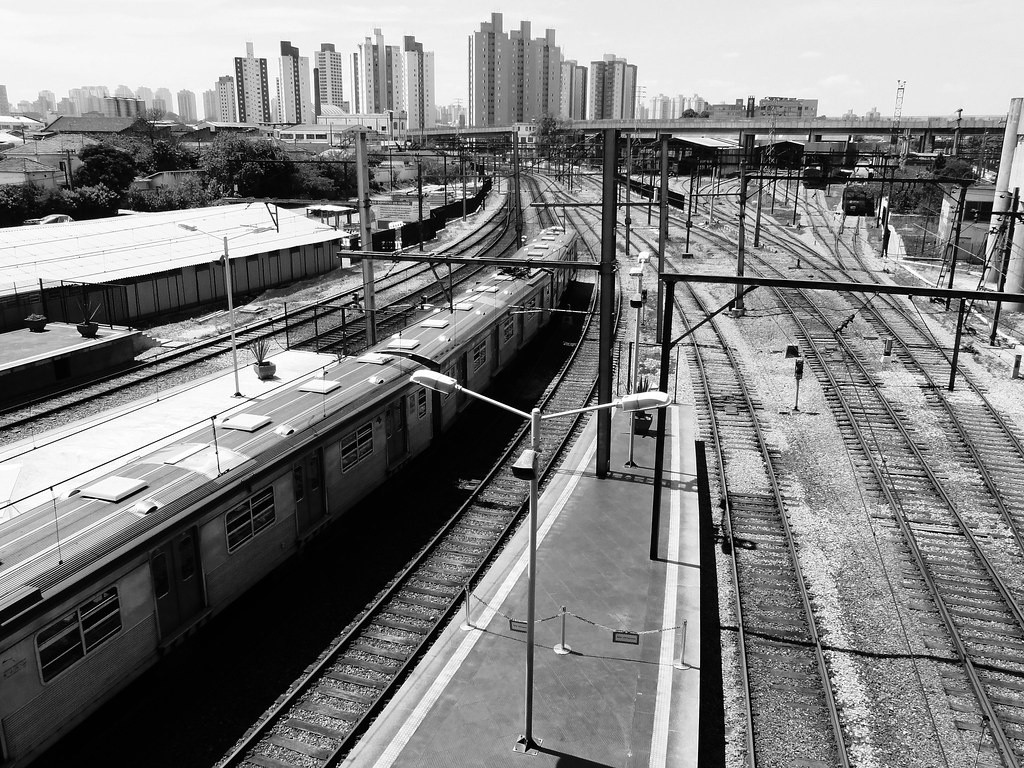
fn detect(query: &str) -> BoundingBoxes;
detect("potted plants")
[247,337,276,379]
[25,314,47,332]
[76,295,103,337]
[628,377,653,436]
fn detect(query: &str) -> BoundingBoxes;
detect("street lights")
[179,224,277,398]
[406,370,672,754]
[625,251,651,469]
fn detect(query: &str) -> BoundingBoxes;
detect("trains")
[843,156,874,215]
[1,226,579,768]
[802,162,828,190]
